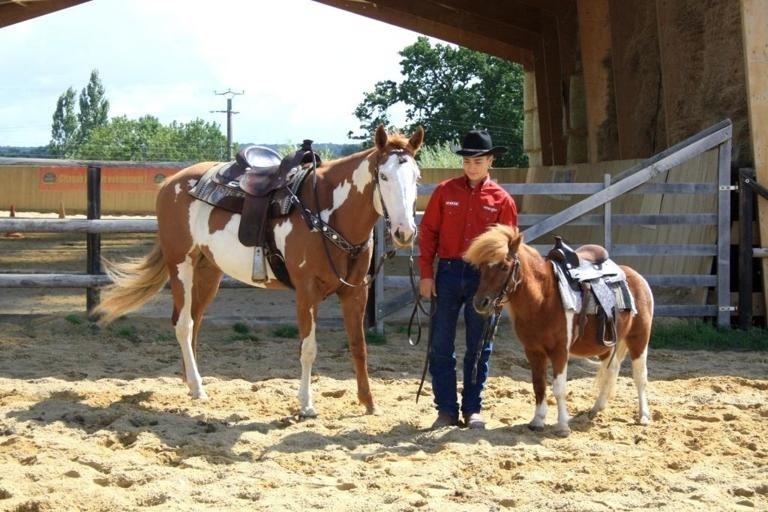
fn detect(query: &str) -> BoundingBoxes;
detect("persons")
[415,130,518,429]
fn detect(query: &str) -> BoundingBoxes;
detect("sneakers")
[465,413,485,429]
[433,414,458,427]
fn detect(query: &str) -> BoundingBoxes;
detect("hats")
[450,130,508,157]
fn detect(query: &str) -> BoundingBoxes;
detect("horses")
[462,222,655,438]
[86,124,425,418]
